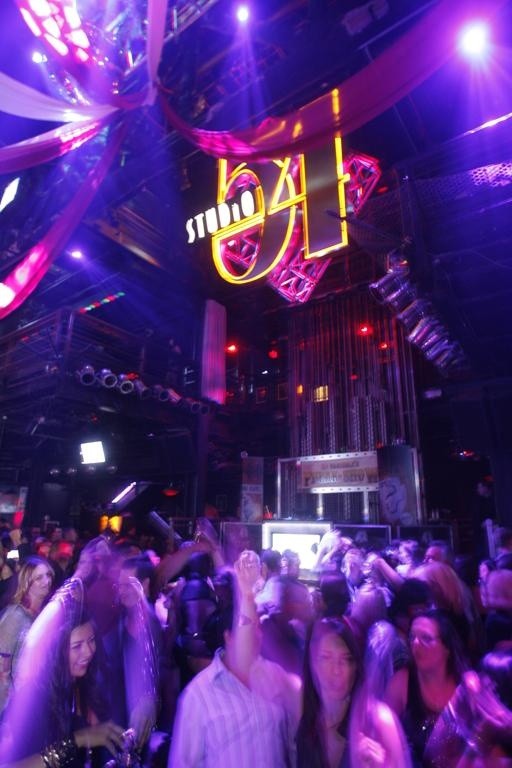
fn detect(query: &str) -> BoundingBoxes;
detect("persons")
[1,499,512,768]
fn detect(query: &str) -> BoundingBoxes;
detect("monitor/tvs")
[77,438,108,467]
[262,522,334,580]
[108,481,137,505]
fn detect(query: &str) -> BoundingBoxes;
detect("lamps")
[367,261,468,379]
[71,361,210,418]
